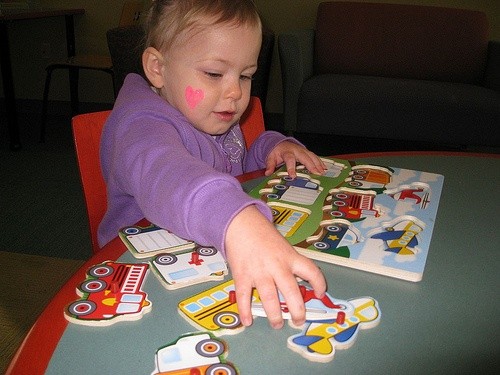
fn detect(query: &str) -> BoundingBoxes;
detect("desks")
[0,9,85,152]
[5,151,500,375]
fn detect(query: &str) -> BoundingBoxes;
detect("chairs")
[71,96,268,254]
[38,0,145,143]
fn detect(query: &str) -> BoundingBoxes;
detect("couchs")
[278,1,500,154]
[106,24,275,114]
